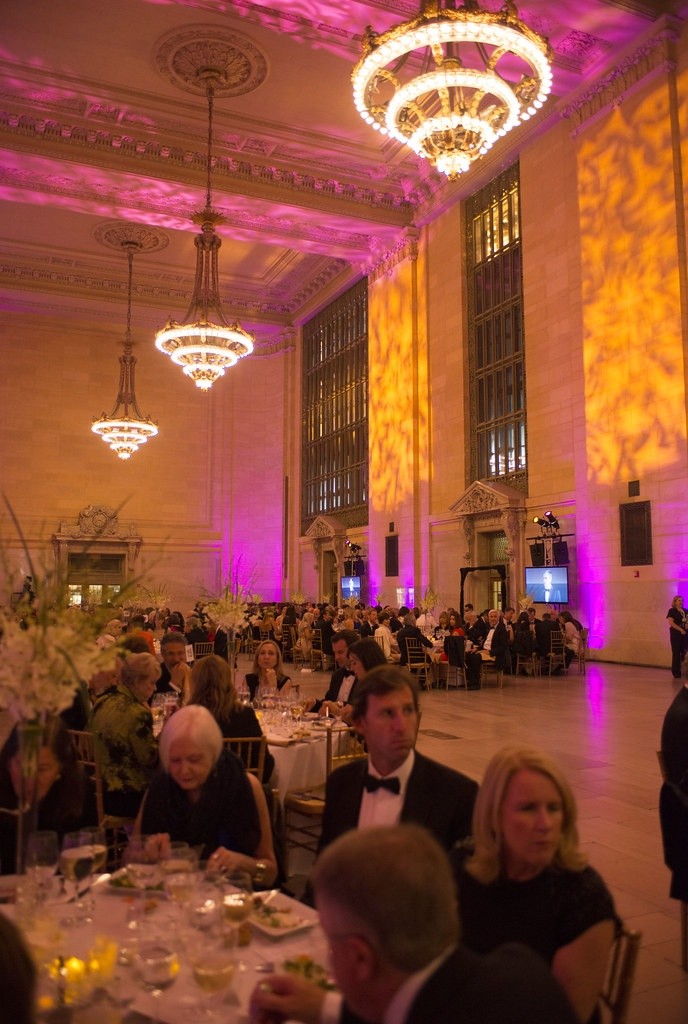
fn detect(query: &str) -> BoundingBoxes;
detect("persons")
[462,744,618,1024]
[18,576,33,608]
[0,910,37,1024]
[119,705,278,892]
[313,666,481,909]
[543,568,553,602]
[666,595,688,678]
[250,824,580,1024]
[0,718,99,875]
[18,601,388,818]
[660,650,688,971]
[194,600,583,690]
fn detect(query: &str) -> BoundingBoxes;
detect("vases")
[16,722,45,933]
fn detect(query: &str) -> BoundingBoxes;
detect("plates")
[102,861,205,897]
[247,890,322,937]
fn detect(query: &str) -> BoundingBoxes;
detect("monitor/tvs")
[525,566,569,604]
[340,576,361,600]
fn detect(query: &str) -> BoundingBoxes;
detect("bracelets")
[253,859,267,886]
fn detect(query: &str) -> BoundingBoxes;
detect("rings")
[213,852,219,860]
[220,865,227,873]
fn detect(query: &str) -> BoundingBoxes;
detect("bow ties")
[530,622,533,625]
[490,627,495,629]
[345,670,355,678]
[365,773,400,795]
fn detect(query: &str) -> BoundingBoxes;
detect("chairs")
[56,623,643,1024]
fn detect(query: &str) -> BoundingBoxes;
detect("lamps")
[88,220,172,463]
[345,540,361,555]
[150,23,270,393]
[533,511,560,537]
[350,0,554,185]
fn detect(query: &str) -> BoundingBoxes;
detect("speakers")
[529,543,548,566]
[552,541,570,565]
[344,561,353,576]
[353,560,365,576]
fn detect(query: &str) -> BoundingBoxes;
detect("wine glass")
[235,686,305,738]
[18,830,252,1023]
[150,691,179,736]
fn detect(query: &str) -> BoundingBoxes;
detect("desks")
[149,692,349,840]
[0,860,345,1024]
[387,635,473,686]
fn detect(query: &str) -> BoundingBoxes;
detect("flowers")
[421,592,436,613]
[0,495,151,721]
[316,594,333,605]
[287,591,307,605]
[343,595,362,609]
[205,551,267,636]
[252,595,263,605]
[514,589,535,612]
[142,582,173,610]
[374,593,385,603]
